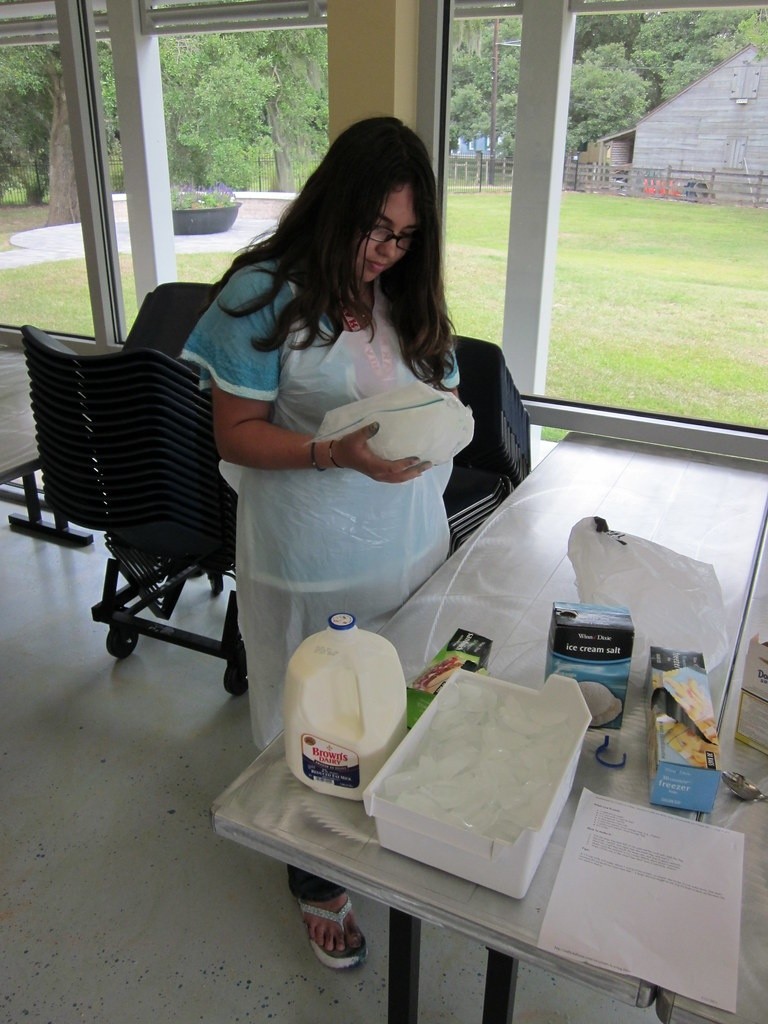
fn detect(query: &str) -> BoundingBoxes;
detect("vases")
[174,202,242,235]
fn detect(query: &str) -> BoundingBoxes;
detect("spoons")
[720,770,768,800]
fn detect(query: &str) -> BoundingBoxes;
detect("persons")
[210,113,462,969]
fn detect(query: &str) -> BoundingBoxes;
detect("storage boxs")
[407,627,493,729]
[363,668,593,898]
[644,646,722,814]
[734,633,768,756]
[544,601,635,730]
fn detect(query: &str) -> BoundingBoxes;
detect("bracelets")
[329,440,344,468]
[311,442,327,472]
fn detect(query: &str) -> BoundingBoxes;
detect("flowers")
[169,182,238,210]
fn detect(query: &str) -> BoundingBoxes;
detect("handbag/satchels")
[567,514,728,681]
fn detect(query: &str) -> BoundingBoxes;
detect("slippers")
[297,892,369,968]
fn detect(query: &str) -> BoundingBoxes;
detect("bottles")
[282,611,406,801]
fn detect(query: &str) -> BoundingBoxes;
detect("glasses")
[367,226,417,251]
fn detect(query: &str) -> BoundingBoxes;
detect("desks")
[211,430,768,1024]
[0,345,95,546]
